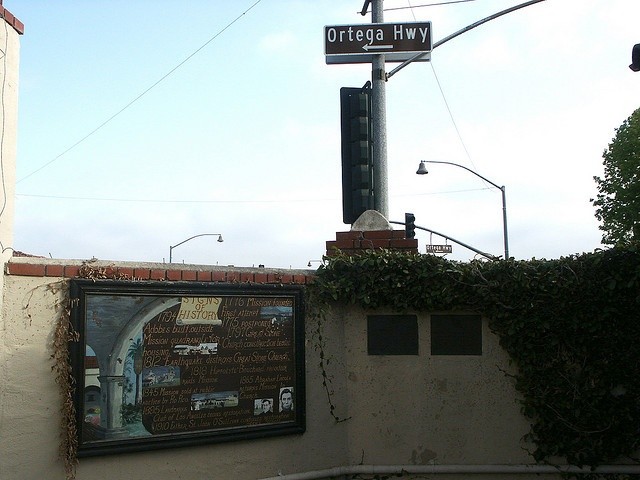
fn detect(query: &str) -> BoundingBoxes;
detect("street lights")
[416,160,509,262]
[169,232,225,264]
[307,259,328,272]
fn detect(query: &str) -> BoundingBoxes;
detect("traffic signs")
[324,21,433,53]
[426,245,452,254]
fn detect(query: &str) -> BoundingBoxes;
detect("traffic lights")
[406,213,415,239]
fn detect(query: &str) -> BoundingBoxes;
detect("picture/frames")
[68,280,306,459]
